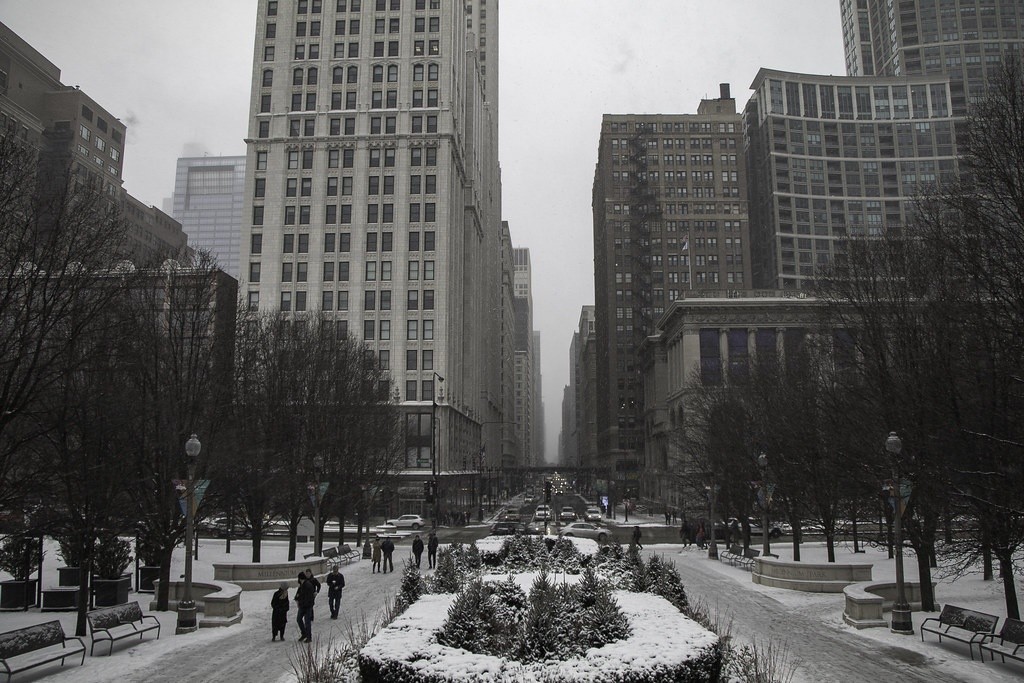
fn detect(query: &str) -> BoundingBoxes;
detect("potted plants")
[0,504,197,612]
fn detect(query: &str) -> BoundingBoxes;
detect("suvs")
[384,514,425,530]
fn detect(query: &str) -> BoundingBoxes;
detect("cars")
[559,506,577,521]
[522,473,574,504]
[195,514,272,540]
[525,522,547,535]
[534,504,553,522]
[584,508,602,523]
[713,515,785,539]
[490,509,527,536]
[554,522,613,540]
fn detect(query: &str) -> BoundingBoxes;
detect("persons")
[427,534,438,568]
[696,519,705,550]
[663,507,677,525]
[632,526,643,550]
[327,566,345,619]
[294,569,321,643]
[372,536,381,573]
[731,520,740,545]
[440,510,471,526]
[747,522,752,545]
[271,583,289,641]
[380,536,394,573]
[412,535,424,567]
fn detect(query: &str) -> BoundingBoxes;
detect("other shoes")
[272,637,275,642]
[331,614,337,620]
[281,636,285,641]
[299,633,306,640]
[304,638,312,643]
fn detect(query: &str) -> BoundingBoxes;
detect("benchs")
[720,545,779,573]
[921,604,1000,661]
[304,544,361,572]
[0,620,87,683]
[85,601,160,656]
[979,618,1024,663]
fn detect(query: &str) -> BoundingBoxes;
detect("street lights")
[884,431,915,635]
[708,462,719,560]
[758,451,770,555]
[432,371,445,537]
[174,431,202,636]
[477,421,517,521]
[311,452,325,556]
